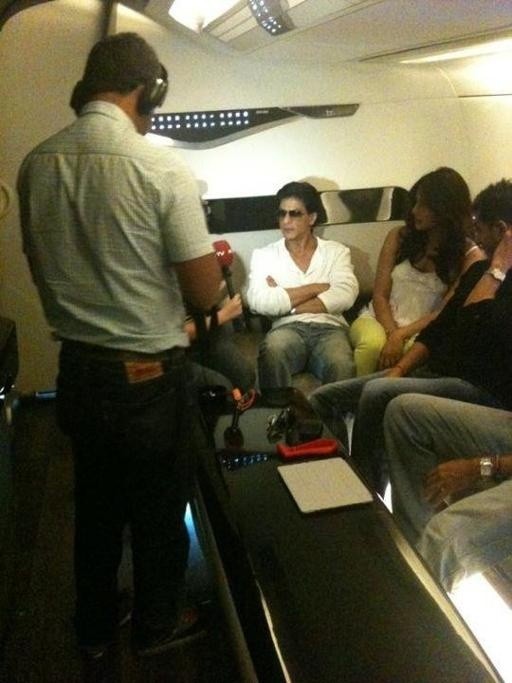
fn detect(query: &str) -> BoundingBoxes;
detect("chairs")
[130,607,211,655]
[77,598,132,660]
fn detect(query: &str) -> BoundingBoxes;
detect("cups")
[273,207,309,218]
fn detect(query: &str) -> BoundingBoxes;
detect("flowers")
[213,240,242,329]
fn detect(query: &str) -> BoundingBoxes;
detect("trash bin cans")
[484,264,507,282]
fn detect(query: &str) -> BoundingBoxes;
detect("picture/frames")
[183,385,504,683]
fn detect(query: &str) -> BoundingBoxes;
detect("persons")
[14,29,225,667]
[245,180,360,419]
[308,176,512,547]
[382,390,512,602]
[346,165,490,378]
[177,271,257,401]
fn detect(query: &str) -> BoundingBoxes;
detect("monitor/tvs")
[275,456,374,516]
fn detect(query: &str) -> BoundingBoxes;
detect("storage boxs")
[200,290,377,457]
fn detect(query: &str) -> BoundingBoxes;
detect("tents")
[70,64,169,112]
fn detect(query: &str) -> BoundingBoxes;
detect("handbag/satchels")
[479,455,495,484]
